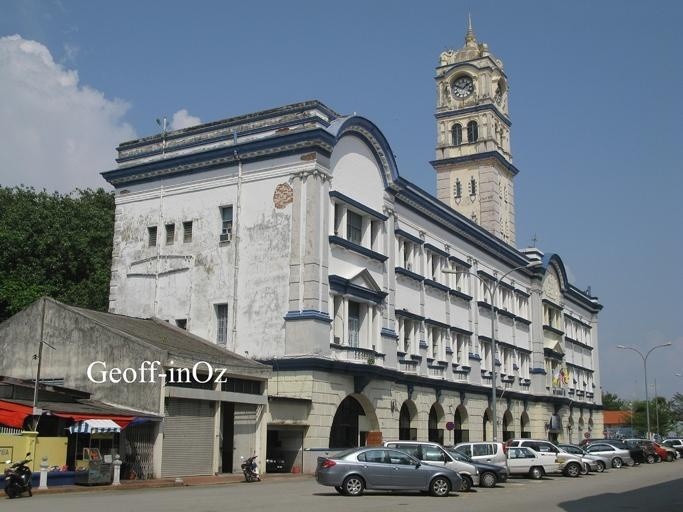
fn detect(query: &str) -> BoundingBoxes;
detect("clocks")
[495,83,502,105]
[452,76,473,101]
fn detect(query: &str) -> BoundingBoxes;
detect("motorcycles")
[240,450,261,482]
[4,452,32,499]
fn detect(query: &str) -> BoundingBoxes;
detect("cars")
[266,448,286,473]
[315,438,683,497]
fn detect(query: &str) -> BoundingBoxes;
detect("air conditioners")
[219,233,231,243]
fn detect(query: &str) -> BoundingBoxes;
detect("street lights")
[441,261,544,442]
[617,343,672,440]
